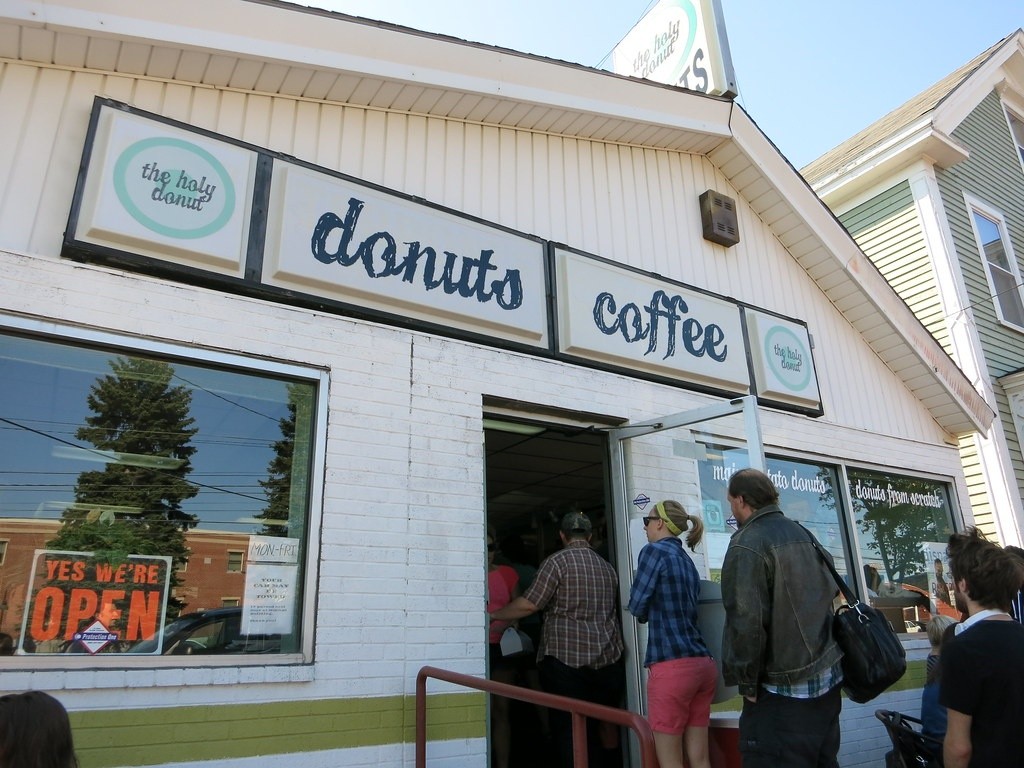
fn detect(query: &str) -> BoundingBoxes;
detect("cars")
[124,605,282,657]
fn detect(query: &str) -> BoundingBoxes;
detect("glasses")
[487,543,496,552]
[643,516,668,526]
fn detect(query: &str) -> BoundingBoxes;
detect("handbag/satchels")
[500,627,534,656]
[793,520,906,704]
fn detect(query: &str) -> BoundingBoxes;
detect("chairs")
[875,708,944,768]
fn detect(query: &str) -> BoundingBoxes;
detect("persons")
[721,469,844,768]
[1004,545,1024,624]
[935,559,951,613]
[15,626,36,652]
[66,599,120,654]
[0,633,14,656]
[628,501,718,768]
[927,616,958,678]
[486,522,521,768]
[0,691,78,768]
[921,622,961,740]
[938,526,1024,768]
[489,512,624,768]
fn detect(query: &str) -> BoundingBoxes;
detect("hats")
[557,511,591,535]
